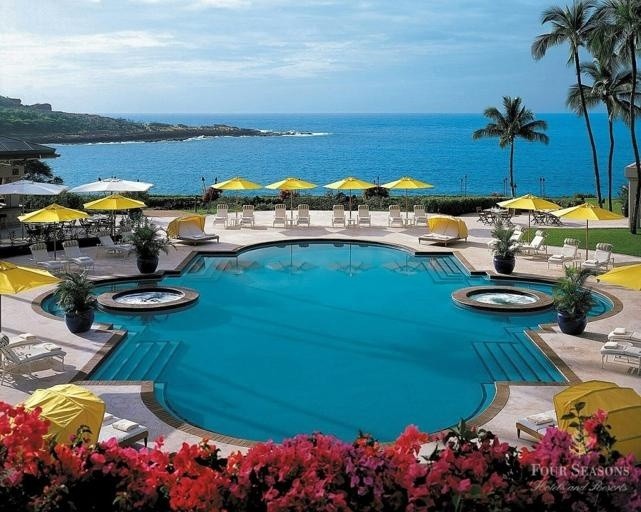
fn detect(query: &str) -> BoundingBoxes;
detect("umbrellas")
[17,203,91,261]
[497,193,562,247]
[67,177,154,236]
[0,180,68,241]
[323,176,378,224]
[265,178,318,226]
[211,176,263,224]
[0,260,62,332]
[597,263,640,291]
[550,202,625,260]
[83,193,146,237]
[379,176,435,224]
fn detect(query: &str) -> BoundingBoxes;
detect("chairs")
[25,235,131,270]
[516,230,549,259]
[168,216,219,245]
[417,217,468,246]
[476,205,563,227]
[214,203,425,228]
[34,386,149,449]
[548,238,580,269]
[516,409,558,443]
[582,243,615,273]
[490,226,522,253]
[600,327,641,375]
[2,331,68,384]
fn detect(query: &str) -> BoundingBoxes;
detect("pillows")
[531,414,551,426]
[110,419,140,433]
[552,255,564,258]
[101,412,113,420]
[585,258,598,265]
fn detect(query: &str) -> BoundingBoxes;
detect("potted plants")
[51,270,105,333]
[550,263,598,334]
[488,224,522,274]
[120,216,179,272]
